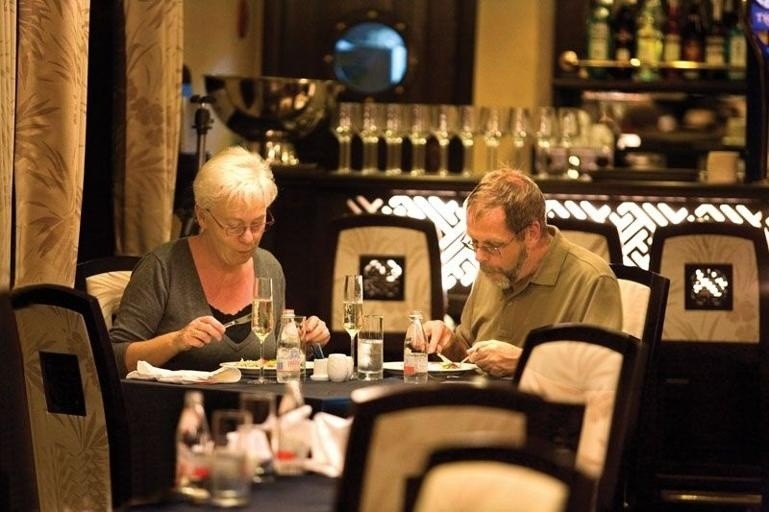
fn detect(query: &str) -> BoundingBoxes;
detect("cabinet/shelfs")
[547,0,768,185]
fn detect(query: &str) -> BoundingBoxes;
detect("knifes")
[224,313,251,329]
[434,349,452,365]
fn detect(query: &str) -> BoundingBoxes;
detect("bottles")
[587,1,750,70]
[403,308,428,383]
[273,382,309,479]
[173,389,211,504]
[627,110,746,171]
[276,308,300,384]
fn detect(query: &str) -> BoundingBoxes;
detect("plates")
[381,360,477,377]
[220,361,314,376]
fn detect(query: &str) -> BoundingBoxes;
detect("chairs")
[610,266,670,359]
[2,284,131,511]
[512,324,644,512]
[545,218,625,266]
[332,384,548,510]
[643,222,769,511]
[74,255,142,330]
[408,446,597,511]
[315,214,443,364]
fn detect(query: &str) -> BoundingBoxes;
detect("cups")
[311,352,354,381]
[708,152,740,186]
[279,316,306,382]
[356,314,385,382]
[207,387,278,509]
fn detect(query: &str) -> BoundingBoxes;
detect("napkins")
[125,360,242,384]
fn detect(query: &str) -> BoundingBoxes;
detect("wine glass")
[342,275,363,381]
[203,68,586,186]
[252,277,275,388]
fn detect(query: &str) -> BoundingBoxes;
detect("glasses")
[461,231,518,259]
[204,205,277,237]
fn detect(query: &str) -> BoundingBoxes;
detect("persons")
[108,144,331,381]
[410,164,623,381]
